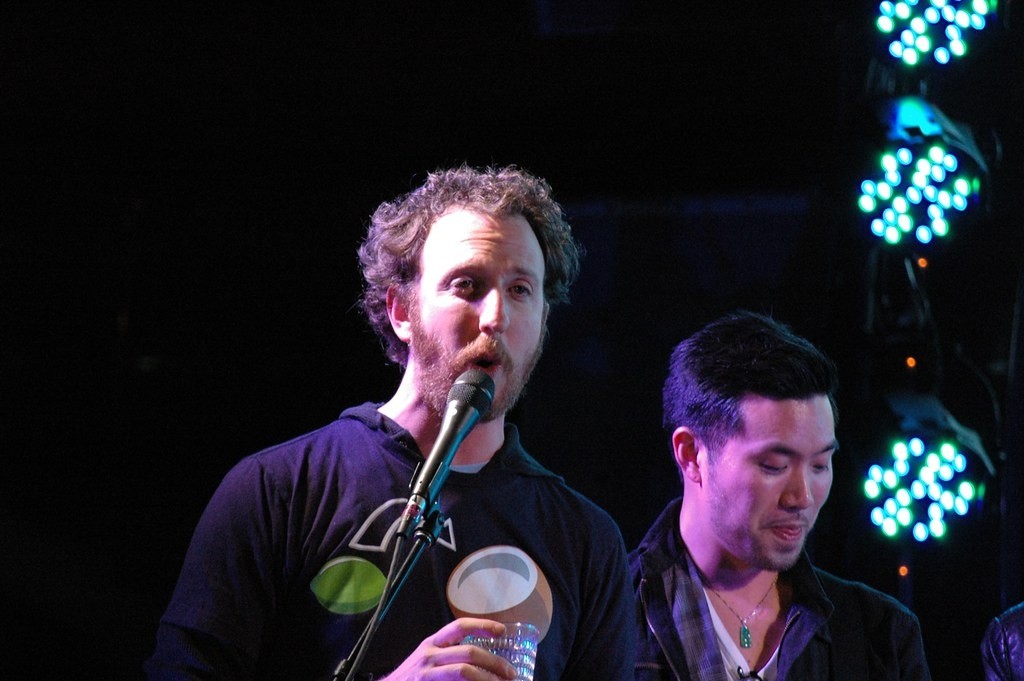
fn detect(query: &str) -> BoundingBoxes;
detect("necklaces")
[704,572,780,650]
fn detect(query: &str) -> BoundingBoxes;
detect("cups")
[459,623,539,681]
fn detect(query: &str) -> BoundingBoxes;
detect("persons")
[141,158,636,681]
[628,308,933,681]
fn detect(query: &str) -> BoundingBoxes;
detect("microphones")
[395,368,495,540]
